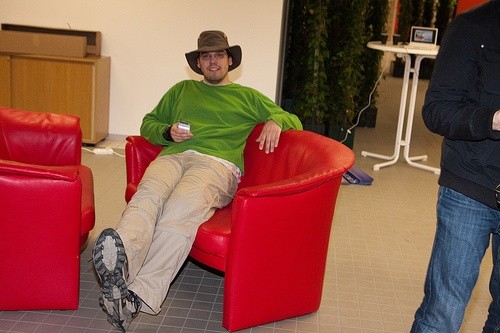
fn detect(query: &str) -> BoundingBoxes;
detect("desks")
[360,41,442,176]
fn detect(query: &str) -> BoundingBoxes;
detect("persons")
[409,0,500,333]
[88,30,303,333]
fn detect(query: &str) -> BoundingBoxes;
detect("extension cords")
[93,148,113,154]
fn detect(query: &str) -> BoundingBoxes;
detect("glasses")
[199,51,228,60]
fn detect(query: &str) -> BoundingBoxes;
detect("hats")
[185,30,242,75]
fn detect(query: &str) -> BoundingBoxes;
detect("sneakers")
[92,227,129,301]
[98,291,142,333]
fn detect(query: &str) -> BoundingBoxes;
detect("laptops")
[404,26,438,51]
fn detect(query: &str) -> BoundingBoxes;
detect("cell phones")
[178,121,191,133]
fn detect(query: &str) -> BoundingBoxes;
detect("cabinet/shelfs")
[0,52,111,147]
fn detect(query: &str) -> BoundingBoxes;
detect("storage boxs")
[0,30,88,57]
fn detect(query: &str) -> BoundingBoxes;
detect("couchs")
[0,106,97,314]
[123,123,355,333]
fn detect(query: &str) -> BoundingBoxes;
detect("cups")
[382,34,400,45]
